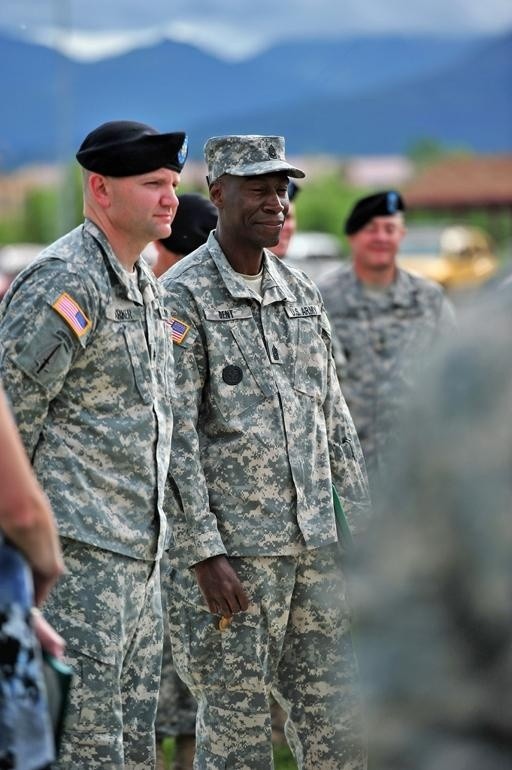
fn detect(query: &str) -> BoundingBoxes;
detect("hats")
[347,188,407,234]
[77,120,191,179]
[203,132,304,186]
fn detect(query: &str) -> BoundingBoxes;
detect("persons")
[0,381,70,770]
[150,192,218,769]
[315,188,456,498]
[0,119,191,770]
[156,132,374,769]
[268,181,302,741]
